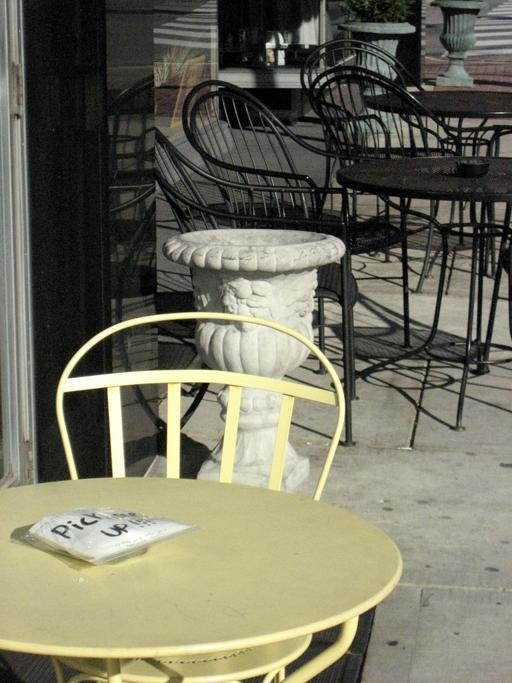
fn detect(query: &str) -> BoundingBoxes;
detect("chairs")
[106,75,155,316]
[183,77,349,251]
[152,124,350,397]
[308,65,507,156]
[50,311,346,683]
[304,38,454,153]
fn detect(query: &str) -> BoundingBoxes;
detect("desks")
[366,88,512,290]
[342,154,511,434]
[1,478,403,683]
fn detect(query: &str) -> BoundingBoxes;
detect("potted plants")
[338,0,417,96]
